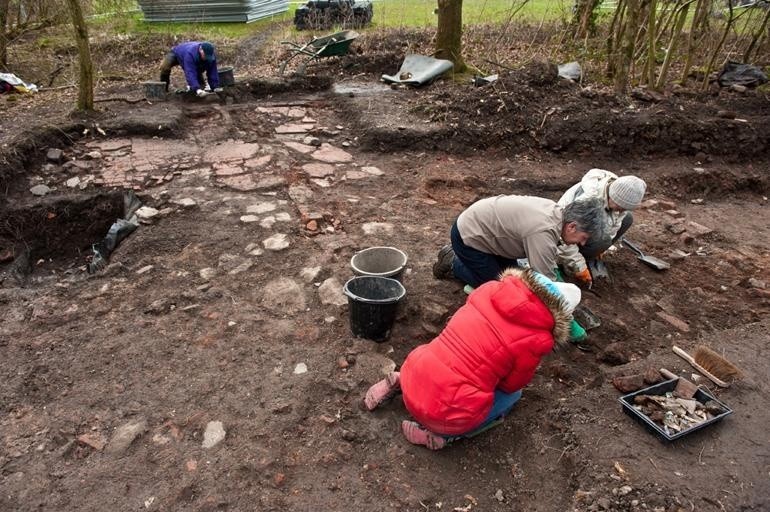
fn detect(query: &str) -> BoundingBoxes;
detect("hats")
[201,41,215,60]
[608,175,647,210]
[553,281,582,313]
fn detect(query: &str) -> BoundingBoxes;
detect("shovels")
[554,268,601,331]
[622,239,671,270]
[589,281,602,299]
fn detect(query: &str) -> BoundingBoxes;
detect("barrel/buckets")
[343,277,405,342]
[216,67,234,86]
[143,82,166,101]
[351,245,408,275]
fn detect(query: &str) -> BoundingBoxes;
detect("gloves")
[569,319,588,342]
[196,88,206,98]
[574,269,592,284]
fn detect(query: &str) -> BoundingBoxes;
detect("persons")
[361,264,584,454]
[157,37,229,99]
[434,192,612,288]
[555,165,650,292]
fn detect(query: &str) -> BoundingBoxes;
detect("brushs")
[672,345,738,388]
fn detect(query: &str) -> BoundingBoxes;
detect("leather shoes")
[364,371,400,411]
[432,245,454,279]
[402,420,455,450]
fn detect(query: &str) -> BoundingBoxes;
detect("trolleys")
[280,29,361,61]
